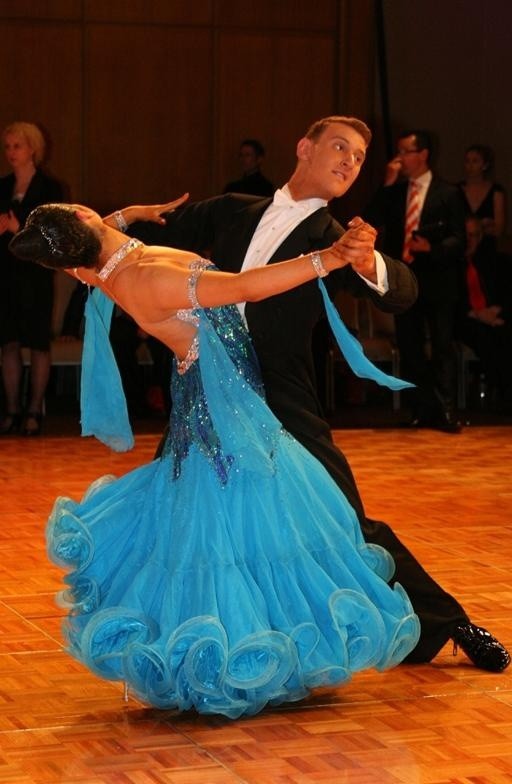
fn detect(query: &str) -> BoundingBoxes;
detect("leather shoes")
[433,409,463,431]
[407,415,431,428]
[451,623,512,672]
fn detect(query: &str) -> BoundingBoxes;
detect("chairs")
[324,288,401,412]
[21,269,84,418]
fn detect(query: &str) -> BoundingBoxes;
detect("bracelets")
[112,209,132,235]
[309,251,329,282]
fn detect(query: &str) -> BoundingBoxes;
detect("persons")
[446,144,505,308]
[1,119,67,439]
[220,139,275,201]
[459,220,512,424]
[7,185,417,721]
[124,113,512,674]
[361,127,469,436]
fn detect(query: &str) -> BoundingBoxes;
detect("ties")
[402,184,421,266]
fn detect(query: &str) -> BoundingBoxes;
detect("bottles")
[477,373,493,425]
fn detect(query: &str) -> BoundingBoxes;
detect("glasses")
[397,147,423,157]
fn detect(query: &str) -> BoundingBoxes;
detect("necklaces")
[94,236,141,283]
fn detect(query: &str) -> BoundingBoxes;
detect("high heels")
[22,412,43,436]
[0,411,23,434]
